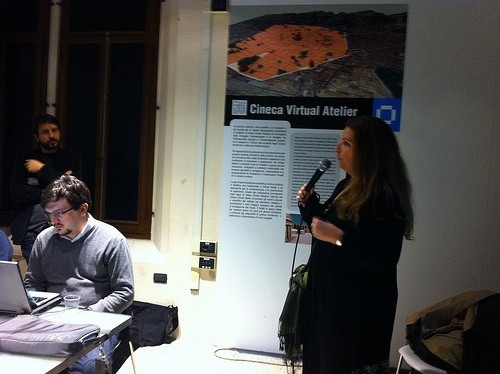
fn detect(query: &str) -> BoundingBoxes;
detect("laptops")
[0,261,62,315]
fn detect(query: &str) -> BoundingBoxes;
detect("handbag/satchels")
[0,314,110,374]
[118,299,178,347]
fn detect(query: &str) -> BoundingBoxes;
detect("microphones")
[305,158,330,192]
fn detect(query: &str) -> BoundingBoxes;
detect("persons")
[24,173,134,374]
[296,117,414,374]
[11,113,73,266]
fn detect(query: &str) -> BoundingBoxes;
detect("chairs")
[396,346,448,374]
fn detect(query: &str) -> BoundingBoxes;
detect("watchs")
[335,231,343,246]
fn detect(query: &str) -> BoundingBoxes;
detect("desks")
[0,306,137,374]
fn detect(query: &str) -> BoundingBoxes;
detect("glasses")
[44,208,75,219]
